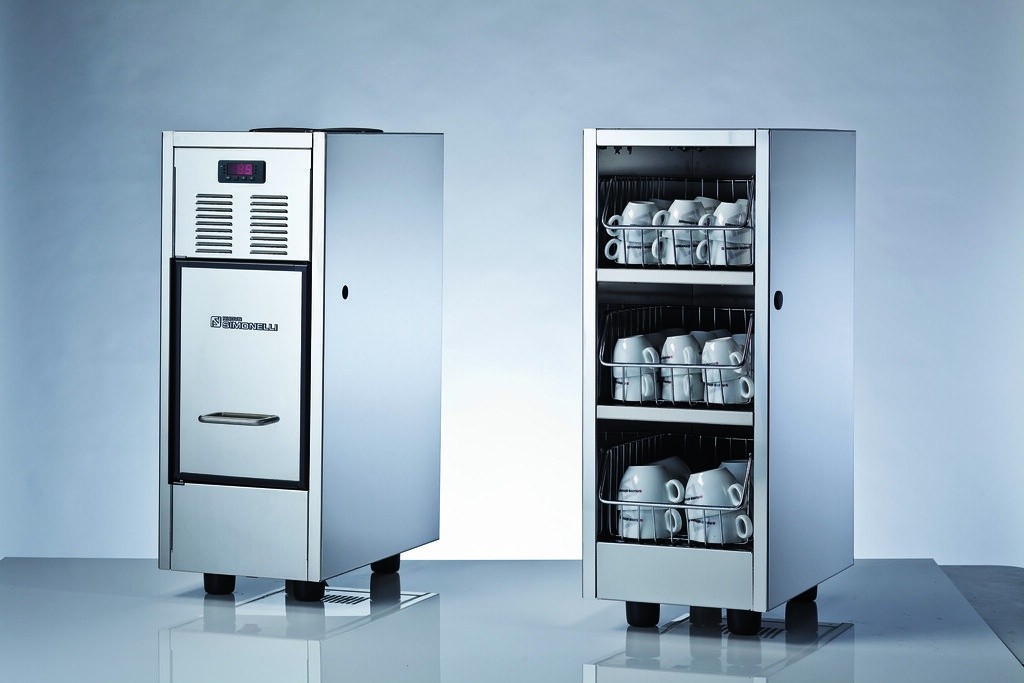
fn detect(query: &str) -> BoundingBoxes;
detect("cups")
[604,197,754,266]
[718,459,751,488]
[617,464,684,510]
[612,329,755,406]
[618,508,682,539]
[683,468,747,520]
[646,451,691,488]
[687,509,753,544]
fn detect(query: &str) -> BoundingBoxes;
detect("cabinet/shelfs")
[583,128,857,636]
